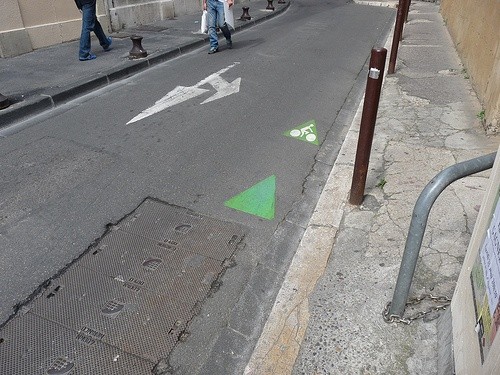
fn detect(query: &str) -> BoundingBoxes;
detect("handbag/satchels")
[200,9,209,34]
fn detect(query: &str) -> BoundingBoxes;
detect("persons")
[73,0,111,61]
[201,0,232,55]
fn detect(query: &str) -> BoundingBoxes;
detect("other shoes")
[104,36,112,51]
[208,46,218,54]
[225,38,232,48]
[78,53,97,61]
